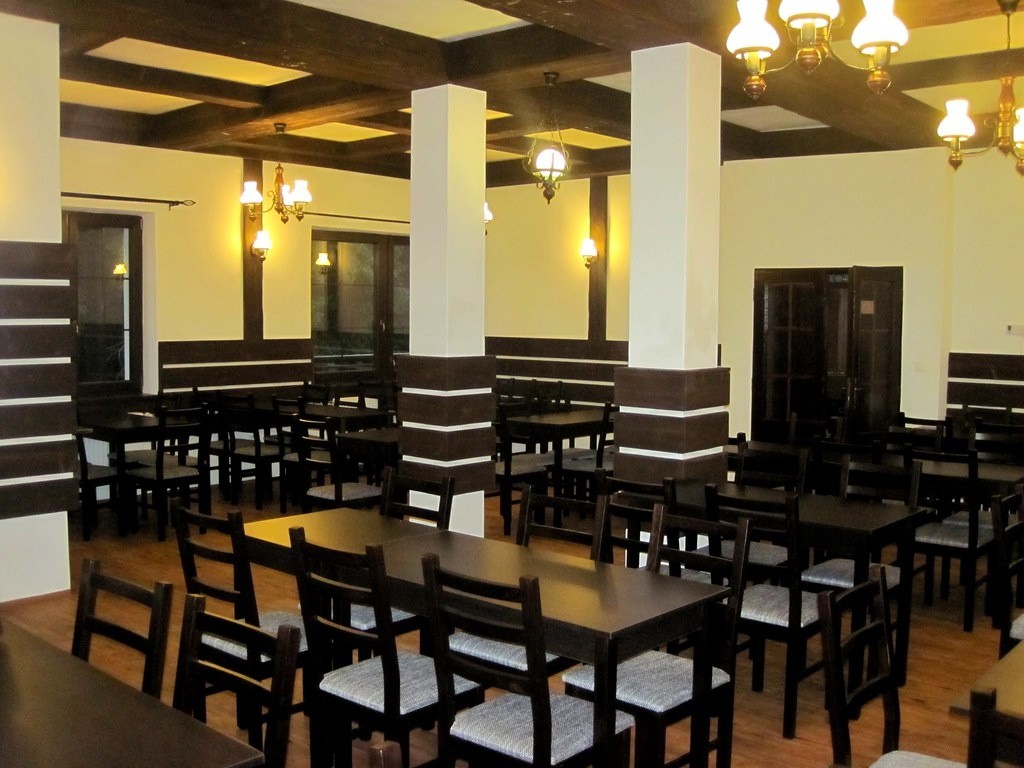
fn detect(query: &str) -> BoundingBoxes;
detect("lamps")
[580,238,597,270]
[520,71,573,204]
[239,123,313,224]
[726,1,908,102]
[251,230,271,261]
[936,0,1024,176]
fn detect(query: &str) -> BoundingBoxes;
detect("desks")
[725,440,1024,632]
[240,507,448,768]
[332,530,735,768]
[257,400,397,509]
[508,411,616,528]
[1,617,267,768]
[613,479,937,740]
[977,640,1024,768]
[77,405,201,539]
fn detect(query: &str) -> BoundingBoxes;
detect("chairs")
[69,376,1023,768]
